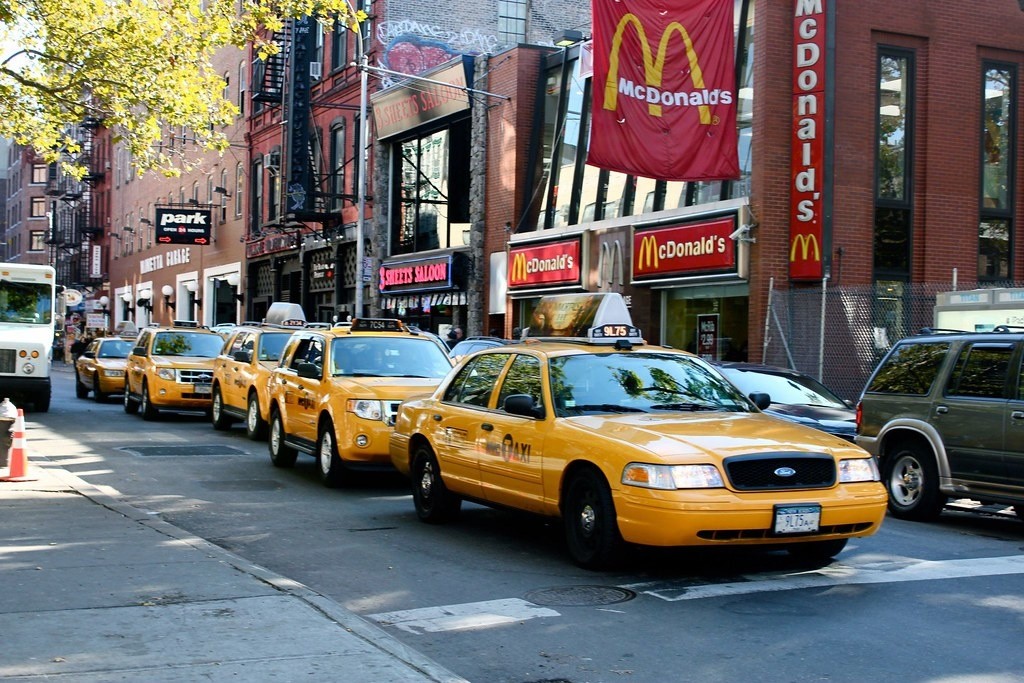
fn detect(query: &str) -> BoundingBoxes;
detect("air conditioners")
[310,61,322,80]
[263,153,280,170]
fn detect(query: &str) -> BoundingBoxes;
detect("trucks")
[0,262,66,411]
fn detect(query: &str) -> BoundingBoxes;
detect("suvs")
[209,302,318,442]
[268,317,459,489]
[438,336,524,363]
[122,319,230,422]
[853,324,1024,521]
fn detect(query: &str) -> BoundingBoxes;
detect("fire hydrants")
[0,397,19,466]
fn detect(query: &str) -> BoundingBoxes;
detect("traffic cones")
[0,408,39,481]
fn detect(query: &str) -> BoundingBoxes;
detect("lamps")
[189,200,217,210]
[123,293,135,317]
[100,295,112,319]
[226,273,244,306]
[139,217,155,228]
[121,227,136,235]
[186,280,203,311]
[162,285,176,313]
[553,30,588,47]
[107,232,122,241]
[140,289,154,315]
[212,185,231,198]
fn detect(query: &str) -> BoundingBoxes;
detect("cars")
[388,292,889,576]
[705,361,860,449]
[76,321,138,402]
